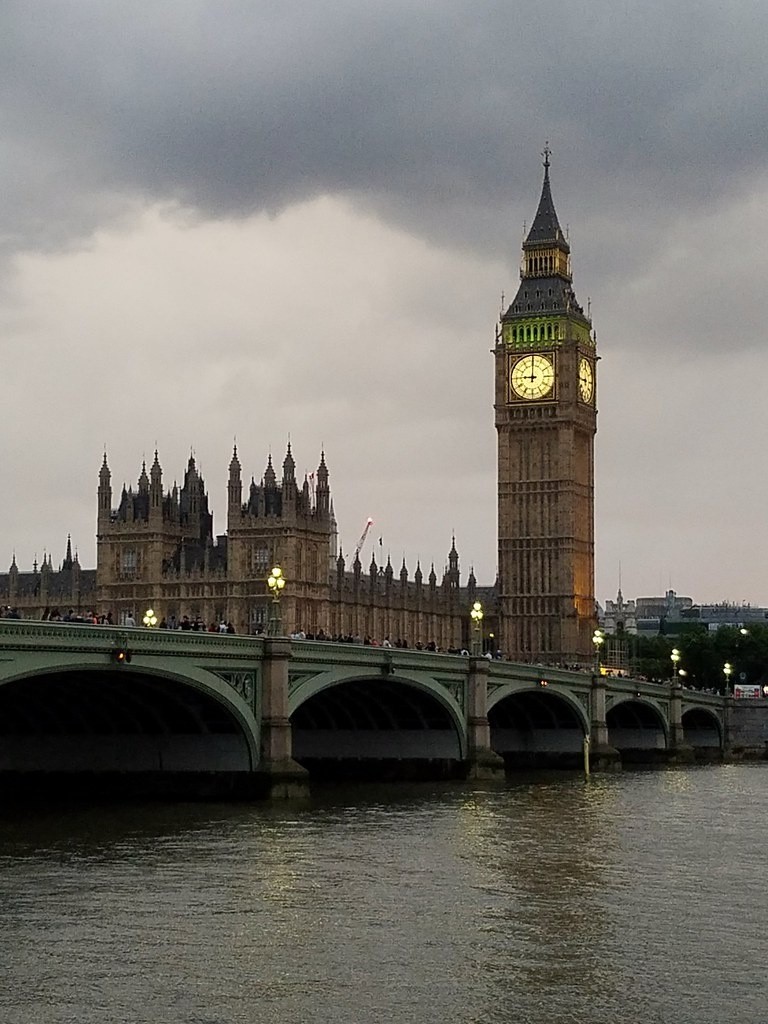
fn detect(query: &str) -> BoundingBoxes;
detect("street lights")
[592,630,604,676]
[470,603,483,657]
[268,566,286,638]
[671,649,681,688]
[723,663,731,696]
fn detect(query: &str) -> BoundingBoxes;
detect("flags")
[379,538,382,545]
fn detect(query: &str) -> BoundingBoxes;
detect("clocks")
[510,355,554,399]
[579,358,593,403]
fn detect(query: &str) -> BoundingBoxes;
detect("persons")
[3,606,264,635]
[484,649,592,673]
[291,629,472,656]
[606,670,720,696]
